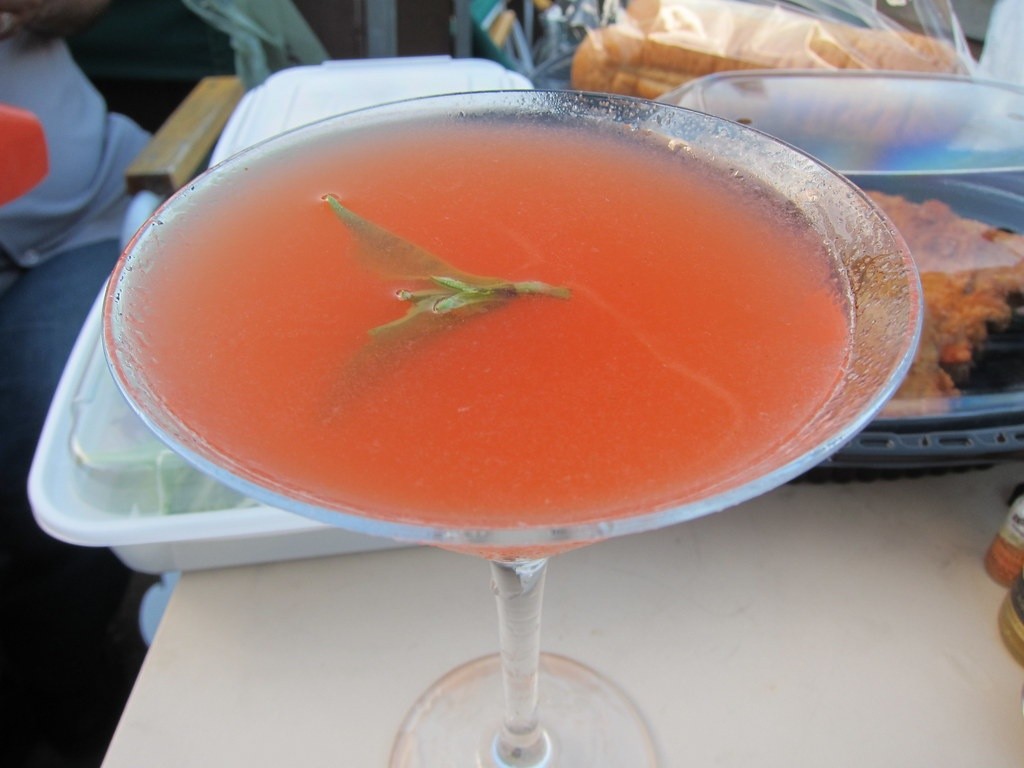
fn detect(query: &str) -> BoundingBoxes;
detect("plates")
[24,278,433,573]
[785,142,1024,483]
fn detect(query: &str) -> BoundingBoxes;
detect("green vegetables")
[326,194,573,350]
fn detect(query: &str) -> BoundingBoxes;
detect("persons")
[0,0,329,768]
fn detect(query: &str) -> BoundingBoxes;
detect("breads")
[567,0,971,148]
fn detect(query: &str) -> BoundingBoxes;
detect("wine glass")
[102,89,924,768]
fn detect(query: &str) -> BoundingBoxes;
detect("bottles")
[998,567,1024,666]
[986,493,1024,587]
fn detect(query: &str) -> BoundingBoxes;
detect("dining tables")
[102,444,1024,768]
